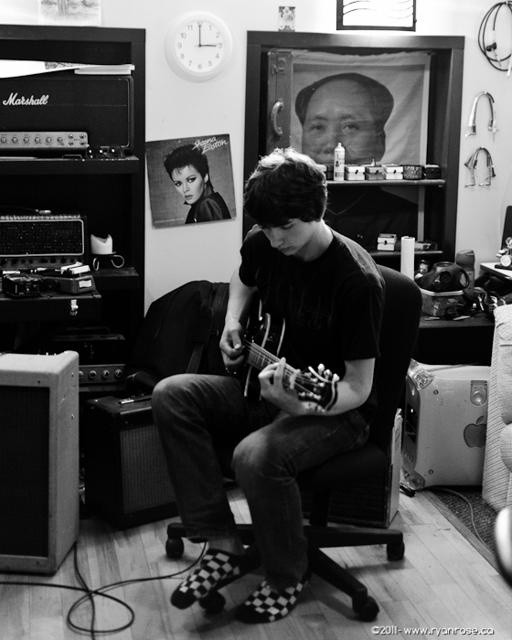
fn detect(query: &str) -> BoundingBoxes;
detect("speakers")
[1,351,79,575]
[88,394,181,533]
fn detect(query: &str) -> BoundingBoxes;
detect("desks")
[418,312,495,364]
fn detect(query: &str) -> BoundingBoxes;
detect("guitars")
[216,297,340,413]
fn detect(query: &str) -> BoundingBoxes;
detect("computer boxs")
[402,360,489,495]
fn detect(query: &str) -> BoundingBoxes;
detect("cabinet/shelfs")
[0,25,146,316]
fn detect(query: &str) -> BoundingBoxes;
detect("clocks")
[166,11,232,80]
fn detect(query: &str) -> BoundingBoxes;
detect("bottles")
[333,141,345,182]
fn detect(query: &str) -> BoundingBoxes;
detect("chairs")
[167,263,417,623]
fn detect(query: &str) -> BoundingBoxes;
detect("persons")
[294,72,418,255]
[149,146,385,624]
[163,144,232,225]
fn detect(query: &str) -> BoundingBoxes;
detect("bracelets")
[307,402,315,415]
